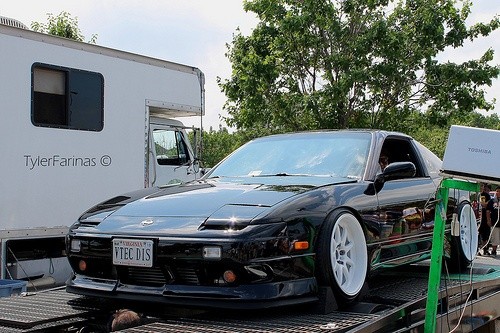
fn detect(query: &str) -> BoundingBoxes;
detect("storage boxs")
[0,278,27,297]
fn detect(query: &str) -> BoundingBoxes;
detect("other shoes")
[484,250,490,256]
[490,251,497,257]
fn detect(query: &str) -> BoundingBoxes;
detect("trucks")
[0,15,206,288]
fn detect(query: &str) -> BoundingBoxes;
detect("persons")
[378,149,389,171]
[476,185,500,258]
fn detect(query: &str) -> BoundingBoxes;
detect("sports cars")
[64,128,483,314]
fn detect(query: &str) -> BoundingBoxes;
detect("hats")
[381,148,391,158]
